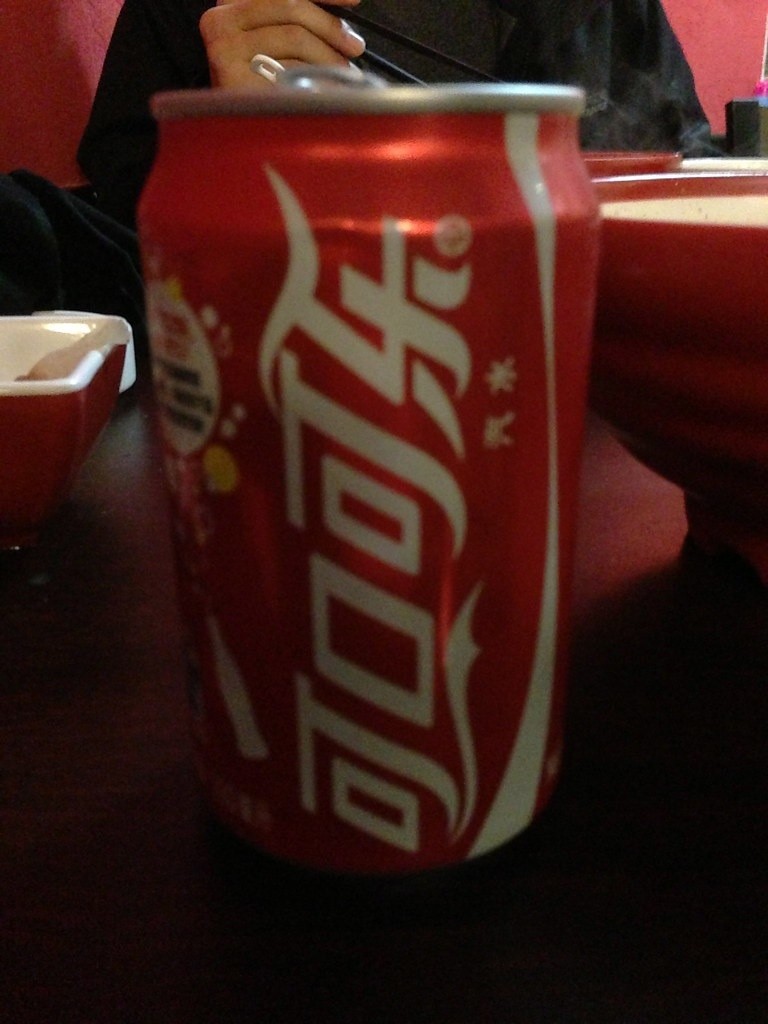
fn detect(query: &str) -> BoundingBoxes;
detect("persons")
[72,0,724,232]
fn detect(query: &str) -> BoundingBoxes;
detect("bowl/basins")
[592,173,768,587]
[583,152,683,180]
[0,310,125,547]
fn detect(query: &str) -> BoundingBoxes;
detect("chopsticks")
[319,6,507,90]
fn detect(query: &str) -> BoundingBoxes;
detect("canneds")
[137,85,599,869]
